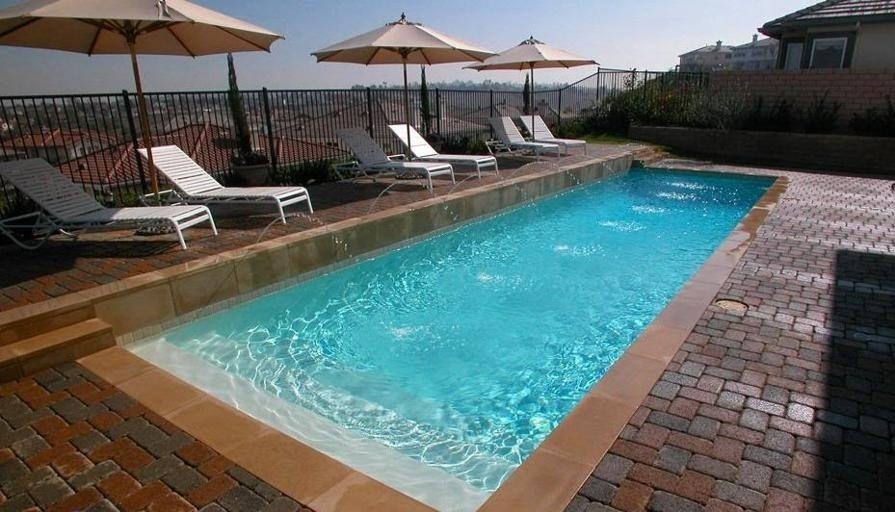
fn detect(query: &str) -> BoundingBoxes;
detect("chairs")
[487,113,587,162]
[333,121,500,195]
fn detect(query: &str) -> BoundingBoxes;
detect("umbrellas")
[0,2,284,235]
[463,37,603,156]
[311,12,499,180]
